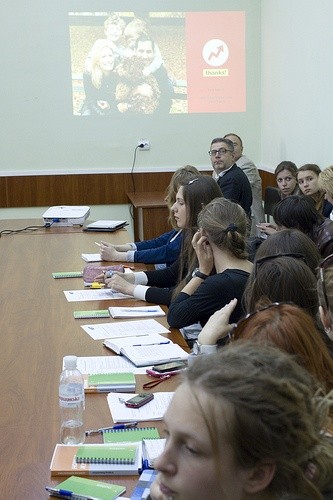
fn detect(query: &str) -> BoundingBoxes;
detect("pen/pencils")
[103,271,110,277]
[45,487,94,500]
[94,242,100,246]
[128,310,157,312]
[85,422,138,435]
[133,341,170,346]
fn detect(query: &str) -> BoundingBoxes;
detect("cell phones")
[153,361,186,373]
[145,366,188,377]
[125,392,154,408]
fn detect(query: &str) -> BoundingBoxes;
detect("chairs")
[264,187,281,222]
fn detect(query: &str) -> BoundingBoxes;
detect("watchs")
[192,339,218,355]
[191,267,209,280]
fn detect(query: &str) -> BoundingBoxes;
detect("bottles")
[58,355,86,445]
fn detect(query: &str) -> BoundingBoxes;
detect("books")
[47,218,193,500]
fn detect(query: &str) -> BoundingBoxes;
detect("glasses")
[208,148,230,156]
[319,253,333,311]
[254,253,308,278]
[234,301,294,340]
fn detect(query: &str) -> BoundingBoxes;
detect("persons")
[318,165,333,221]
[99,165,200,262]
[81,15,175,115]
[211,133,265,241]
[166,196,254,350]
[208,138,253,236]
[187,255,332,363]
[227,301,331,416]
[151,338,333,500]
[93,177,222,307]
[295,163,333,219]
[274,161,304,199]
[273,195,333,259]
[254,230,323,281]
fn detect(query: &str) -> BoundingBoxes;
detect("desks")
[0,218,192,500]
[128,192,172,241]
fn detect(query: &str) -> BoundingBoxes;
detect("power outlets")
[138,141,149,151]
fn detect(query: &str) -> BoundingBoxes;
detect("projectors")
[41,205,91,226]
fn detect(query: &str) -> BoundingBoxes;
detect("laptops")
[83,220,127,232]
